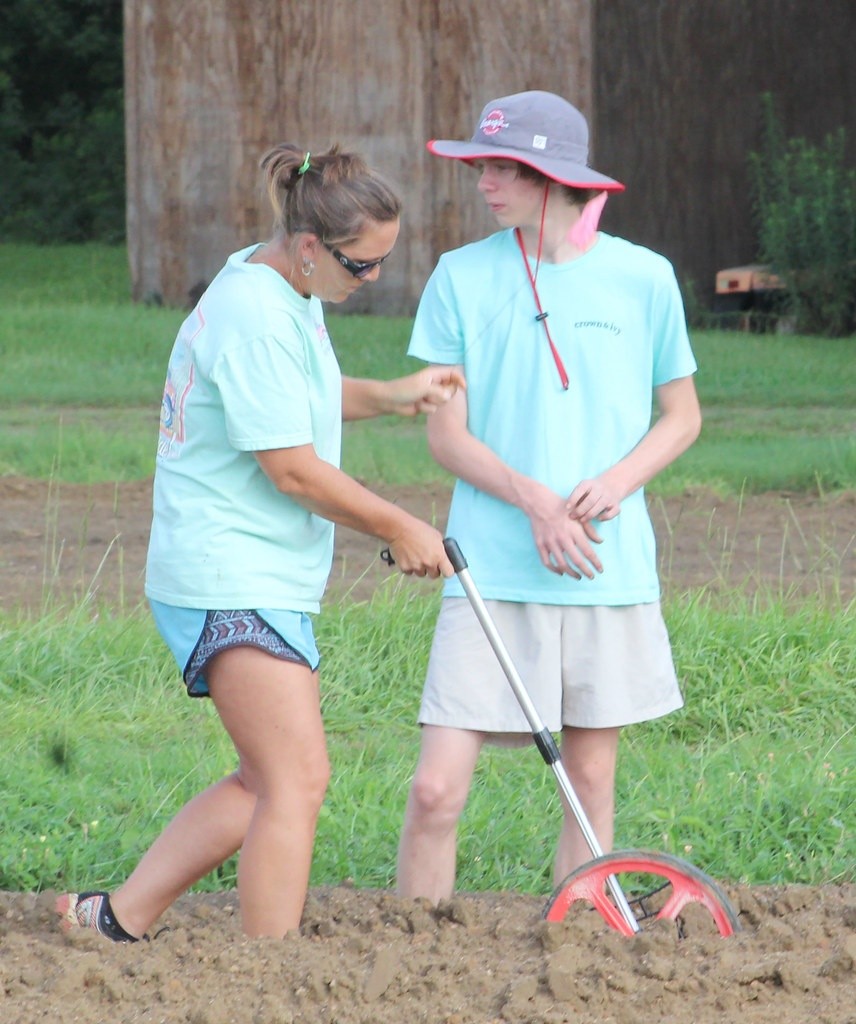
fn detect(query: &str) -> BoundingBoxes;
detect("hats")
[428,92,626,190]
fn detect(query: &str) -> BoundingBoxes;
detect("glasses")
[320,240,392,277]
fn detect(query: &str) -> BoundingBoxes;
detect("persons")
[51,140,464,990]
[388,90,705,932]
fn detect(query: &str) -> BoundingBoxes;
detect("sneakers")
[53,893,168,944]
